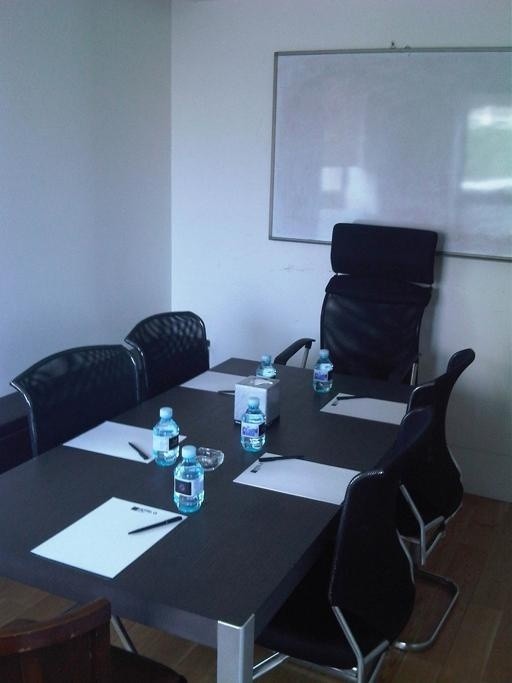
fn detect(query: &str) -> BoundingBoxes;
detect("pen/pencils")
[259,455,304,461]
[219,390,234,392]
[128,515,182,534]
[337,394,371,399]
[129,442,148,458]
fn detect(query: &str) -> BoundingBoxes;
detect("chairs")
[125,312,211,397]
[256,423,450,680]
[376,348,475,649]
[273,222,439,387]
[7,346,143,457]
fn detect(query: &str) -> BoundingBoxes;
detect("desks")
[1,358,428,682]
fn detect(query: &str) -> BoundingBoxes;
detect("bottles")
[152,406,180,466]
[241,396,266,452]
[173,444,205,512]
[255,354,277,380]
[312,348,334,392]
